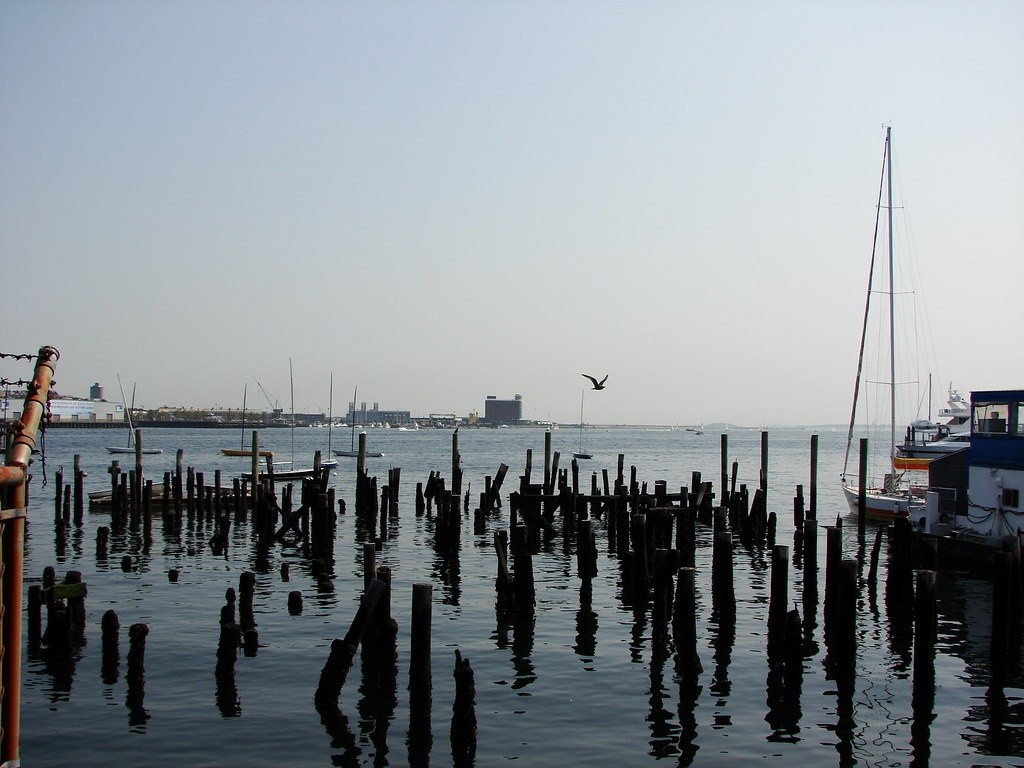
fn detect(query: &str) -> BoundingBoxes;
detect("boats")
[398,426,419,431]
[890,372,981,491]
[551,422,560,430]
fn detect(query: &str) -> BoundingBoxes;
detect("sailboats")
[242,358,327,480]
[106,372,164,454]
[321,371,340,467]
[839,126,925,526]
[572,388,594,459]
[332,386,385,457]
[221,384,273,457]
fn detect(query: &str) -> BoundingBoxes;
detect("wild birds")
[582,373,608,390]
